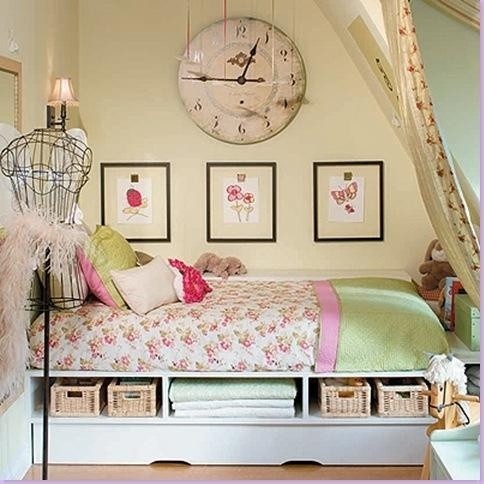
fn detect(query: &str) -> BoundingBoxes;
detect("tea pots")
[0,54,23,135]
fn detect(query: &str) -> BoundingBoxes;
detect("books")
[453,293,480,353]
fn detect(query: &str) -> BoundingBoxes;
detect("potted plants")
[100,160,385,243]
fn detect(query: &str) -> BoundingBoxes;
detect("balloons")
[176,15,307,147]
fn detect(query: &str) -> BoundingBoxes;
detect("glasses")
[47,76,80,131]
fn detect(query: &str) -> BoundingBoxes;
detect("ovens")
[31,269,450,467]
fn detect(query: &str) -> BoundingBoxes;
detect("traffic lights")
[35,223,181,316]
